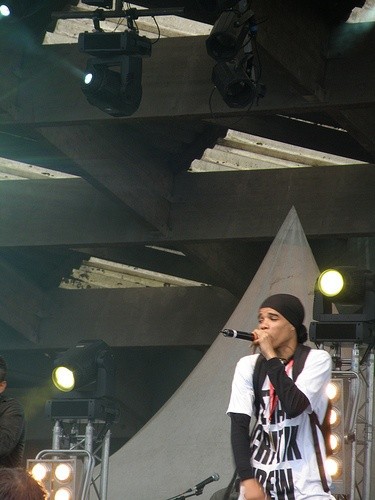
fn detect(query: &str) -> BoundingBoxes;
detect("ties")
[269,360,293,418]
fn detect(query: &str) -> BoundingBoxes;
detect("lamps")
[52,339,112,392]
[79,17,150,116]
[212,57,259,112]
[315,265,373,304]
[206,7,251,63]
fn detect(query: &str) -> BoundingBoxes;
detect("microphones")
[223,328,259,343]
[187,473,220,492]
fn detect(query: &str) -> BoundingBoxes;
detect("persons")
[225,293,335,500]
[0,358,26,468]
[0,466,47,500]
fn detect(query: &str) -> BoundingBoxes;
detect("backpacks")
[253,343,332,456]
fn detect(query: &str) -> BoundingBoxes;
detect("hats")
[260,294,304,331]
